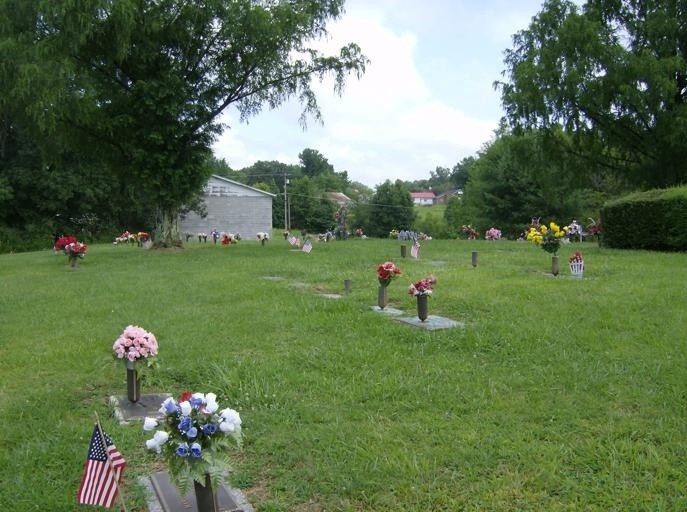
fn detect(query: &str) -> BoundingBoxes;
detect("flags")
[78,423,126,508]
[295,236,300,245]
[411,238,421,258]
[287,233,296,246]
[302,239,313,253]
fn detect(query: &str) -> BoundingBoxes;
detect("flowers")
[144,391,242,495]
[113,325,161,370]
[409,275,437,296]
[389,222,600,257]
[376,262,400,287]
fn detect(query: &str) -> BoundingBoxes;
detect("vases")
[344,280,351,293]
[126,359,140,404]
[472,251,478,267]
[417,296,428,321]
[552,257,559,275]
[193,473,215,512]
[71,256,76,267]
[378,287,387,309]
